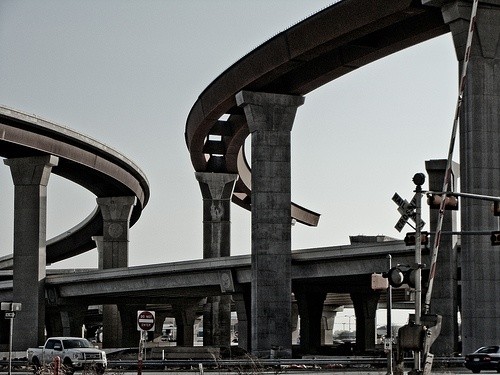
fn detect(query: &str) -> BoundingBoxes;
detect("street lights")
[345,314,354,339]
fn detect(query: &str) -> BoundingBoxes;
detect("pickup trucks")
[27,337,107,375]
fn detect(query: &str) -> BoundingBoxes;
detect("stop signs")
[137,311,155,332]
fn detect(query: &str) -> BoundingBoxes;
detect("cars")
[161,336,173,341]
[464,346,500,373]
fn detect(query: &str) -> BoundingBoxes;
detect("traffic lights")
[491,231,500,246]
[387,267,418,289]
[489,200,500,216]
[404,232,428,246]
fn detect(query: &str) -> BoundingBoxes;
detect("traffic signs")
[5,312,16,319]
